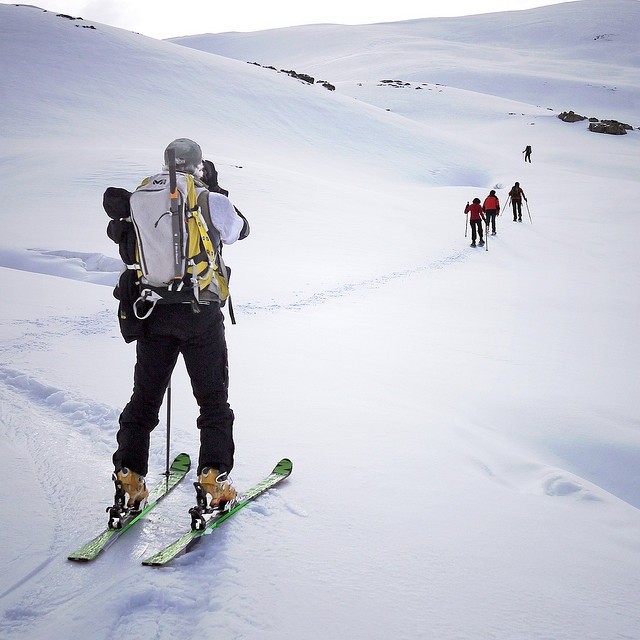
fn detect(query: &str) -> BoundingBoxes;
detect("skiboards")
[68,453,293,567]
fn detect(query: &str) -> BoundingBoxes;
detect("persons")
[522,145,533,164]
[463,197,488,247]
[508,182,528,222]
[112,138,253,510]
[483,189,500,234]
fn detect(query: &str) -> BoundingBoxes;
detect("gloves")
[200,160,222,193]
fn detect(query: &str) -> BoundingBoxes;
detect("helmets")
[164,138,202,174]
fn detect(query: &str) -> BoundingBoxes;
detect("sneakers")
[193,467,238,514]
[112,466,149,514]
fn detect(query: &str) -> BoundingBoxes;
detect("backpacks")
[129,174,237,324]
